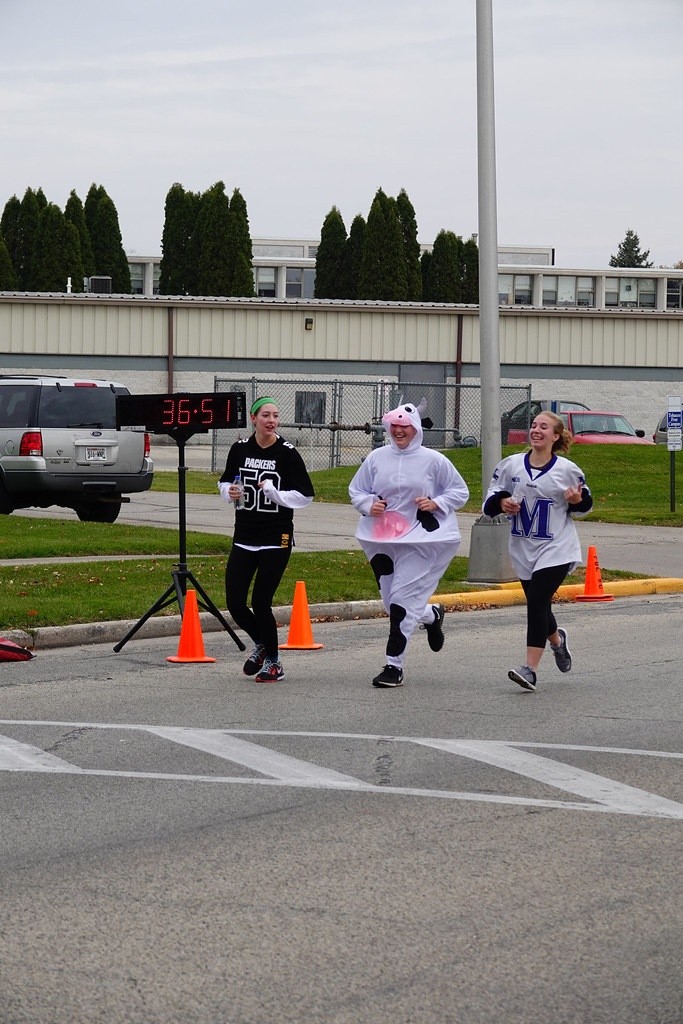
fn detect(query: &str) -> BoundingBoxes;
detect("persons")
[217,396,315,684]
[482,411,593,690]
[349,396,469,687]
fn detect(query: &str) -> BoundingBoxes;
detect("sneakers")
[419,604,445,652]
[243,644,266,676]
[551,628,572,673]
[255,659,285,682]
[372,665,404,686]
[508,666,537,690]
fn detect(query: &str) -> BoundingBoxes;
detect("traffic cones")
[276,580,323,650]
[575,545,616,601]
[166,589,217,663]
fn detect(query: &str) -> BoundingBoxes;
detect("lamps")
[306,318,313,330]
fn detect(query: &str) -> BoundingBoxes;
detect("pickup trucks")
[507,410,658,446]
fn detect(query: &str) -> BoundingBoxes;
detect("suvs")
[0,374,155,523]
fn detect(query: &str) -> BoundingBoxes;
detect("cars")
[501,399,604,450]
[652,411,683,444]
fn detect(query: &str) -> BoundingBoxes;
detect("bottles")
[231,476,244,510]
